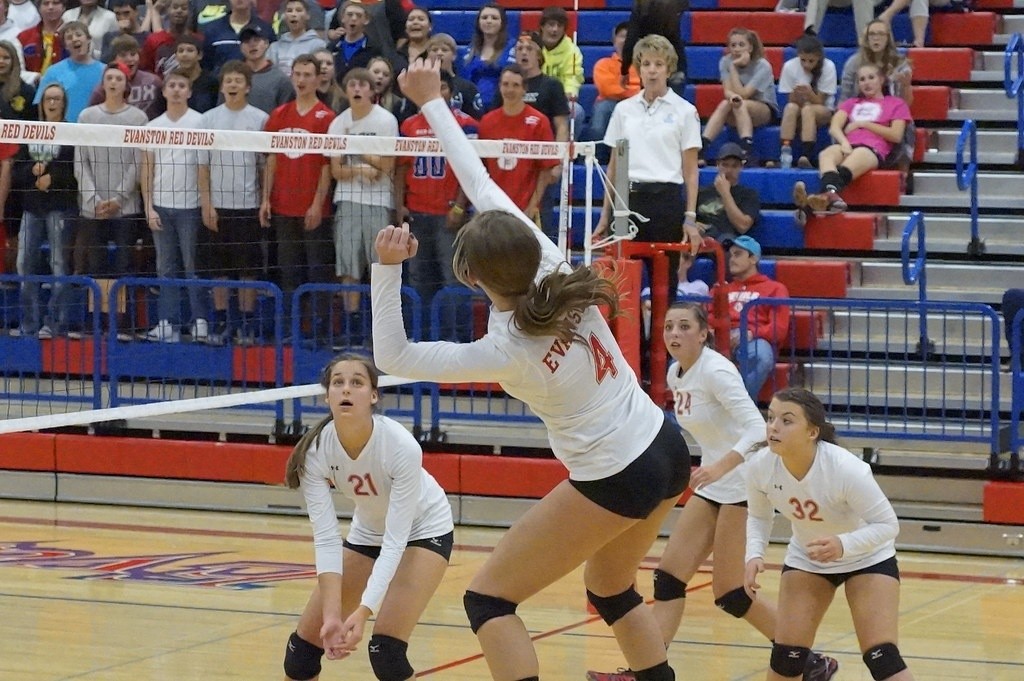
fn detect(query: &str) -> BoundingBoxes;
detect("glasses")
[867,31,888,40]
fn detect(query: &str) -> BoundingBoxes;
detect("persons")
[699,29,780,169]
[793,63,912,226]
[0,1,586,351]
[778,30,838,168]
[371,57,690,681]
[805,0,884,45]
[1002,288,1024,371]
[704,236,790,401]
[695,143,758,280]
[621,0,688,96]
[640,252,709,338]
[837,18,916,172]
[586,302,838,681]
[283,354,455,681]
[744,387,914,681]
[592,35,706,378]
[879,0,974,48]
[588,22,640,141]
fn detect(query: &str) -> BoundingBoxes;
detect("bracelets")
[685,211,696,226]
[449,201,465,214]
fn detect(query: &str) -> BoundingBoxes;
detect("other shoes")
[9,326,22,336]
[766,160,782,169]
[38,326,53,339]
[333,335,364,350]
[807,192,848,214]
[793,181,812,226]
[236,327,254,343]
[117,330,133,341]
[66,328,83,339]
[206,323,229,344]
[799,155,816,169]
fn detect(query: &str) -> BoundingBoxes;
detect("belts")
[628,179,679,196]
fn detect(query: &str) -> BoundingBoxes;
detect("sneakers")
[586,667,637,681]
[137,319,180,342]
[802,653,839,681]
[191,319,209,342]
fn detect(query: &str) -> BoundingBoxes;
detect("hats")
[519,30,545,67]
[613,20,631,36]
[238,23,268,40]
[722,235,761,258]
[718,142,742,159]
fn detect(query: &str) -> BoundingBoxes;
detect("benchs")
[0,0,1010,402]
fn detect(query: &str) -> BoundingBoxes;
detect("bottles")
[781,141,793,169]
[887,61,911,82]
